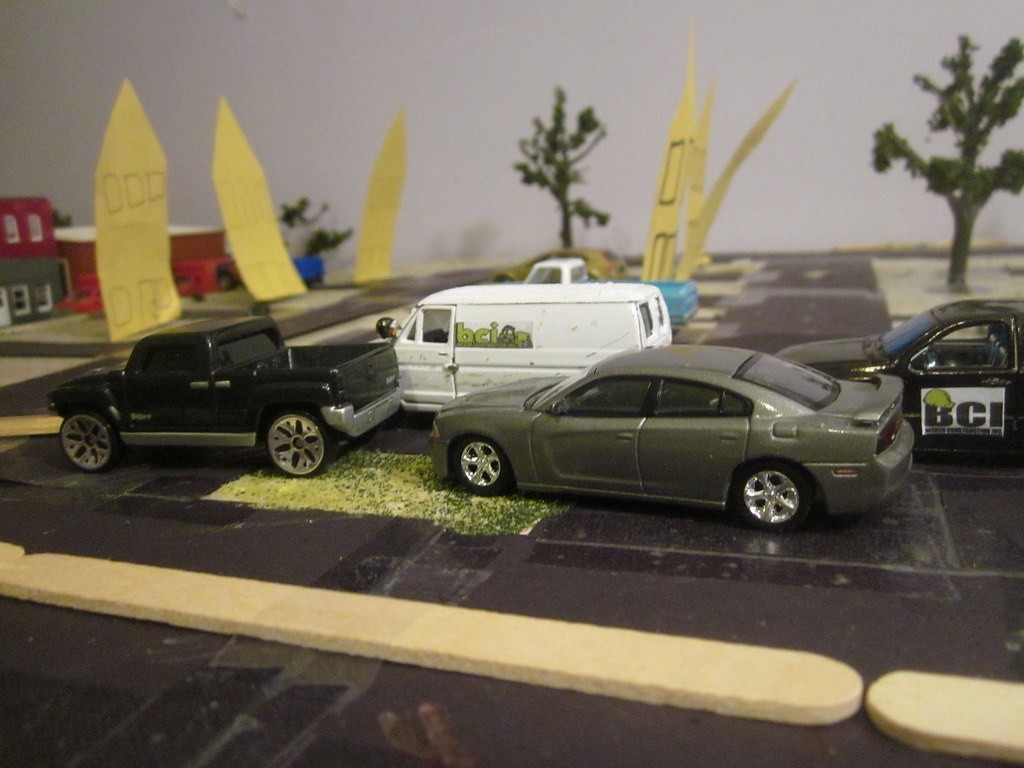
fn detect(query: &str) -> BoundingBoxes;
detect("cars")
[771,298,1024,454]
[490,247,627,290]
[426,343,919,535]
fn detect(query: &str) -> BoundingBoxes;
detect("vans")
[367,283,674,417]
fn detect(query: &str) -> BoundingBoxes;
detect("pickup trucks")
[42,313,404,479]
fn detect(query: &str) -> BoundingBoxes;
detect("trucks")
[525,258,700,326]
